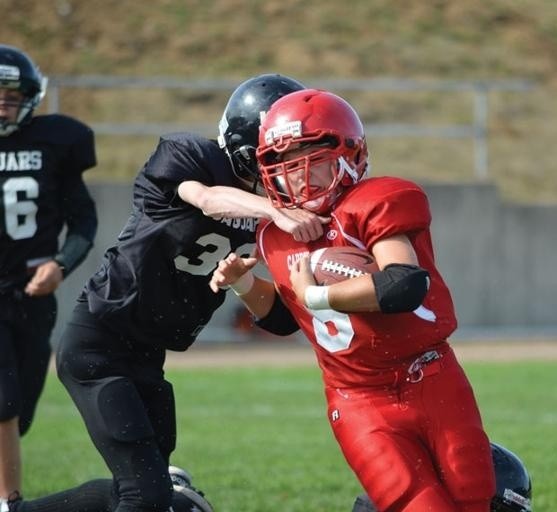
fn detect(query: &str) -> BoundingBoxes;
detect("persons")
[0,70,306,512]
[352,443,531,511]
[208,89,498,511]
[0,47,98,499]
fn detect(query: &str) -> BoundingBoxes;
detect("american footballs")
[309,246,380,286]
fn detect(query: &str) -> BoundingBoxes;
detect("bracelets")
[305,285,333,310]
[229,269,256,296]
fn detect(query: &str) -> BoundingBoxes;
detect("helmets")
[216,68,311,195]
[0,43,48,140]
[255,88,369,216]
